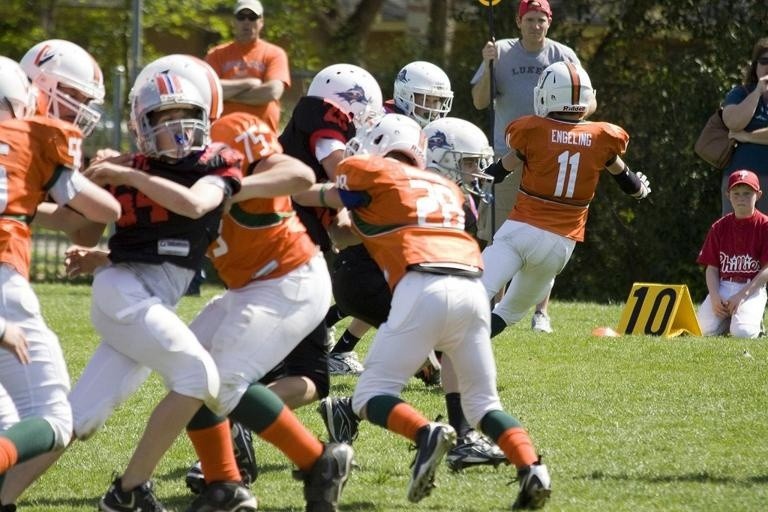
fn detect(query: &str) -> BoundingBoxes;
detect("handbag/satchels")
[692,108,739,171]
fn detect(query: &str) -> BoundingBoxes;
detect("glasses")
[757,57,768,65]
[236,13,263,23]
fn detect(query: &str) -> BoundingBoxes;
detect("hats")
[727,169,761,193]
[232,0,265,16]
[751,36,768,63]
[518,0,554,23]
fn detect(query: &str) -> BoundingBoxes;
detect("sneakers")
[510,453,553,512]
[413,349,442,386]
[98,476,171,512]
[445,426,508,472]
[531,310,553,335]
[316,393,364,448]
[230,415,259,485]
[184,459,207,493]
[301,440,358,511]
[187,477,260,511]
[406,419,458,506]
[323,322,365,374]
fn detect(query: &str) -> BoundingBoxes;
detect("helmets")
[132,74,209,160]
[422,116,496,182]
[392,59,455,115]
[0,54,32,121]
[127,53,225,120]
[532,60,598,120]
[305,62,384,126]
[362,112,425,170]
[20,38,107,114]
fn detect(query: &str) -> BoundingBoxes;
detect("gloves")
[629,171,653,205]
[480,156,494,173]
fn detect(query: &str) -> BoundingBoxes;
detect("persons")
[1,54,243,509]
[696,168,768,340]
[202,2,292,136]
[185,61,553,508]
[2,39,122,509]
[414,61,650,387]
[470,2,597,334]
[721,37,768,217]
[63,54,355,509]
